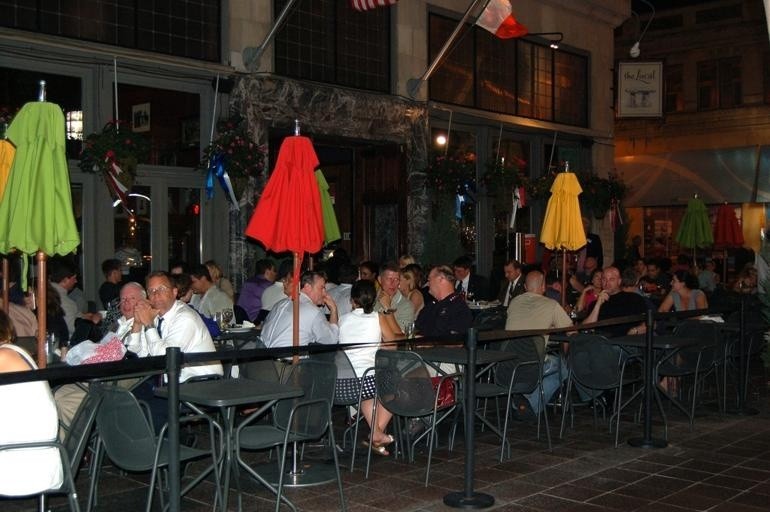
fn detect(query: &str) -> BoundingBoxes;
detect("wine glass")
[222,308,233,331]
[105,301,112,311]
[466,292,475,301]
[34,328,60,351]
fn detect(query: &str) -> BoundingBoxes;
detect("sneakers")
[512,397,536,424]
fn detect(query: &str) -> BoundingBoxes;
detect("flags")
[477,1,529,41]
[352,0,397,12]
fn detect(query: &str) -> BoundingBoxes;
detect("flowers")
[480,152,531,192]
[407,152,478,204]
[76,119,152,176]
[536,169,586,202]
[583,170,624,209]
[194,114,270,179]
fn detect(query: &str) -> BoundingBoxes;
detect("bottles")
[60,347,69,362]
[45,339,54,365]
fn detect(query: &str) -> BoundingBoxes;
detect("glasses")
[147,287,171,295]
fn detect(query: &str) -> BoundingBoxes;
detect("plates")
[226,324,256,333]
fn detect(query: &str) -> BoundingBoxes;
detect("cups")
[404,322,416,351]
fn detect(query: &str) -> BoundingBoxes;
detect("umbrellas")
[712,199,745,282]
[243,118,343,472]
[0,76,82,368]
[675,193,714,268]
[539,159,587,306]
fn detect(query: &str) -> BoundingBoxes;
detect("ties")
[457,280,463,294]
[157,317,164,339]
[508,281,514,300]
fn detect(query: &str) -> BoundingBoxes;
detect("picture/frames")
[617,61,663,118]
[361,191,405,269]
[129,100,153,136]
[177,115,199,145]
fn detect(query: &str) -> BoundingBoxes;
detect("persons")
[0,258,237,449]
[0,309,73,511]
[496,237,718,422]
[734,269,765,294]
[236,253,493,450]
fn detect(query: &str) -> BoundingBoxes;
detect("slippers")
[362,431,395,457]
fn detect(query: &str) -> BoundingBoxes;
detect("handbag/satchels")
[431,376,455,409]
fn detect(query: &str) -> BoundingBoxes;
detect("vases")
[592,202,608,219]
[223,171,249,202]
[102,157,130,197]
[495,185,513,216]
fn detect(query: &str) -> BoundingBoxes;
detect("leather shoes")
[180,433,198,480]
